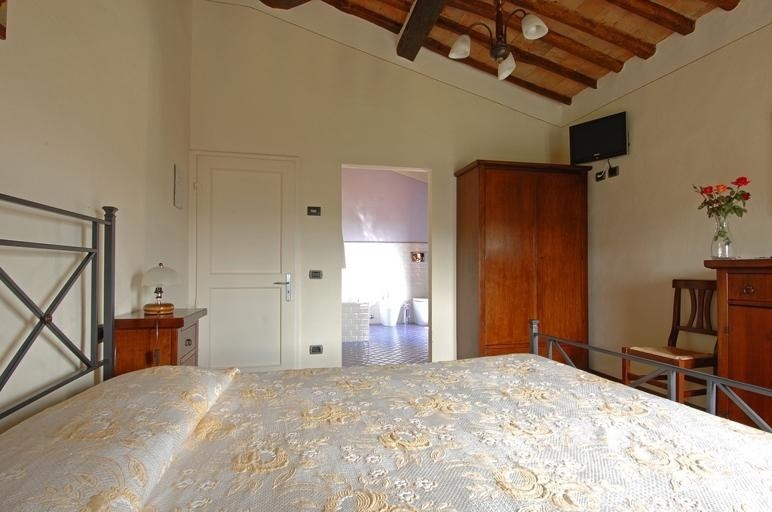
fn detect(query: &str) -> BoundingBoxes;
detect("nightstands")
[114,307,207,375]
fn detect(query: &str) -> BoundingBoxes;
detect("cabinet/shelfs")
[454,159,592,371]
[702,257,771,431]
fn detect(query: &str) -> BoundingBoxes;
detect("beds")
[0,193,770,509]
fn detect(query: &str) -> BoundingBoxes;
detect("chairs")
[622,279,718,403]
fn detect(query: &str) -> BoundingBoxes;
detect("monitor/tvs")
[568,111,629,165]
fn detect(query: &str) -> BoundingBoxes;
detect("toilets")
[411,297,429,327]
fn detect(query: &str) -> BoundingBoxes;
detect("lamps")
[141,263,178,315]
[448,1,550,81]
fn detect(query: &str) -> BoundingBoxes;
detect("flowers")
[689,176,751,244]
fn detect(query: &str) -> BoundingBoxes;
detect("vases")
[710,216,736,259]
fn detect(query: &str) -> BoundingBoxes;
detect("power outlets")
[607,166,618,178]
[595,171,606,181]
